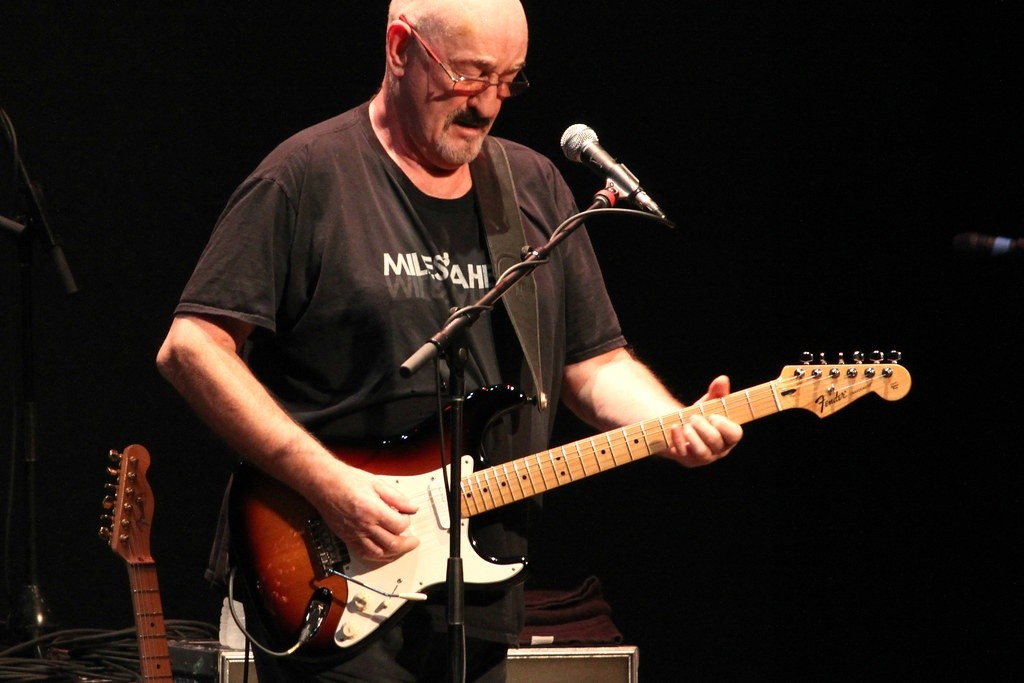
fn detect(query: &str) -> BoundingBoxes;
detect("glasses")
[399,13,531,101]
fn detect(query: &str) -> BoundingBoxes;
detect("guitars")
[225,349,911,666]
[96,443,174,683]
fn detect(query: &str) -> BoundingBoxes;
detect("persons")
[156,0,744,683]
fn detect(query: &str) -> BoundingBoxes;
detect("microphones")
[561,123,667,220]
[955,232,1024,255]
